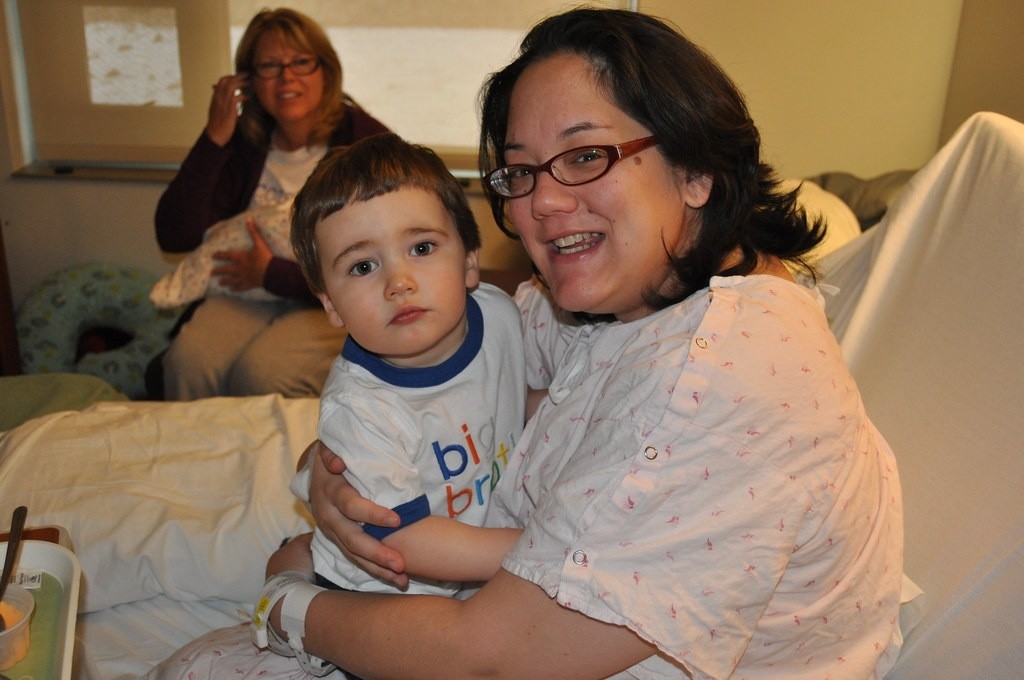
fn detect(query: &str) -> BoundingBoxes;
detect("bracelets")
[247,570,336,678]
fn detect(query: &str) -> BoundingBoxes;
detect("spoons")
[0,506,27,631]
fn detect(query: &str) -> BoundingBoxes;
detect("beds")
[1,112,1024,680]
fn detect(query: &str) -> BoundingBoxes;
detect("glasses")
[482,132,660,199]
[245,51,321,80]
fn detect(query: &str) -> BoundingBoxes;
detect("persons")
[152,8,405,400]
[145,6,924,680]
[289,128,529,601]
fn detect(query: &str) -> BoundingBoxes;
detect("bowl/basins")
[0,584,35,670]
